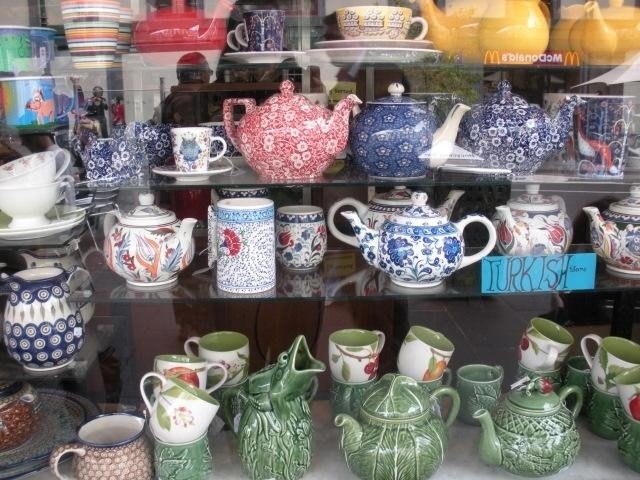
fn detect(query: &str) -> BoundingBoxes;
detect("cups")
[277,206,326,276]
[335,5,428,40]
[0,146,73,229]
[225,19,245,50]
[233,9,283,51]
[0,0,131,130]
[542,91,629,179]
[213,198,278,294]
[168,127,226,172]
[48,411,152,477]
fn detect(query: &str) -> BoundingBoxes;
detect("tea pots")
[583,185,640,278]
[139,312,639,480]
[341,190,496,288]
[100,192,197,289]
[410,1,639,67]
[326,186,468,253]
[2,262,96,373]
[452,78,587,172]
[19,242,106,325]
[136,0,232,67]
[490,185,574,253]
[222,78,359,183]
[356,83,472,181]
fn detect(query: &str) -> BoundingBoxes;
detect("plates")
[306,40,442,63]
[0,206,86,242]
[150,166,238,184]
[0,386,101,478]
[225,51,307,65]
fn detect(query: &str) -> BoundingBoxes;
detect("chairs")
[73,178,122,253]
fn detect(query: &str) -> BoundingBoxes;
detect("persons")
[148,52,419,368]
[24,73,85,144]
[150,51,409,376]
[87,86,110,138]
[17,112,74,179]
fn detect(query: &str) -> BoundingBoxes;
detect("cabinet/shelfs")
[50,51,639,480]
[0,100,132,479]
